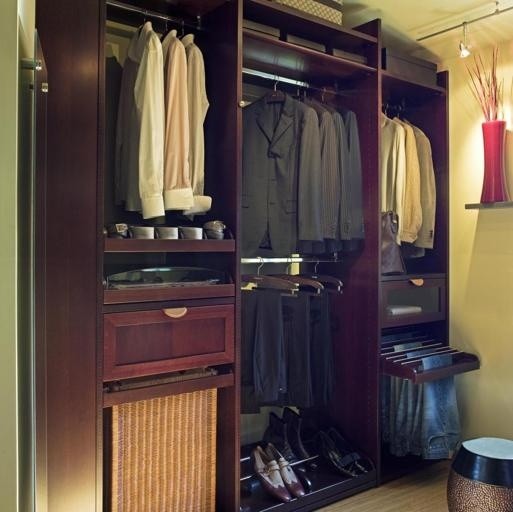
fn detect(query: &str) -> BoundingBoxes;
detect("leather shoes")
[319,428,375,479]
[250,443,306,503]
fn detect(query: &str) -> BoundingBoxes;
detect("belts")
[204,221,226,240]
[106,224,133,239]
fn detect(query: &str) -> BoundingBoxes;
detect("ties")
[130,226,155,239]
[178,226,203,239]
[155,227,179,239]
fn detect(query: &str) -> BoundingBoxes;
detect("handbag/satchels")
[381,211,407,276]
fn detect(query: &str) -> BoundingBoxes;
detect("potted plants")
[465,42,511,204]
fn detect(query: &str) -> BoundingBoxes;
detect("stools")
[446,436,512,511]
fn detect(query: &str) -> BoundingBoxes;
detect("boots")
[263,407,318,473]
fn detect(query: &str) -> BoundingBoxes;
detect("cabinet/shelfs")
[33,1,482,512]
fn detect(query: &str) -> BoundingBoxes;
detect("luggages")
[273,0,344,27]
